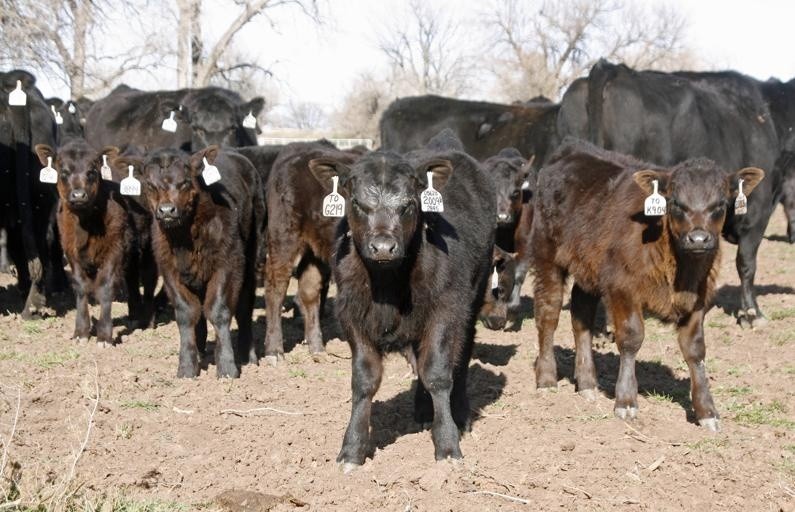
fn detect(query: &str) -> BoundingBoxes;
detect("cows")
[1,58,795,466]
